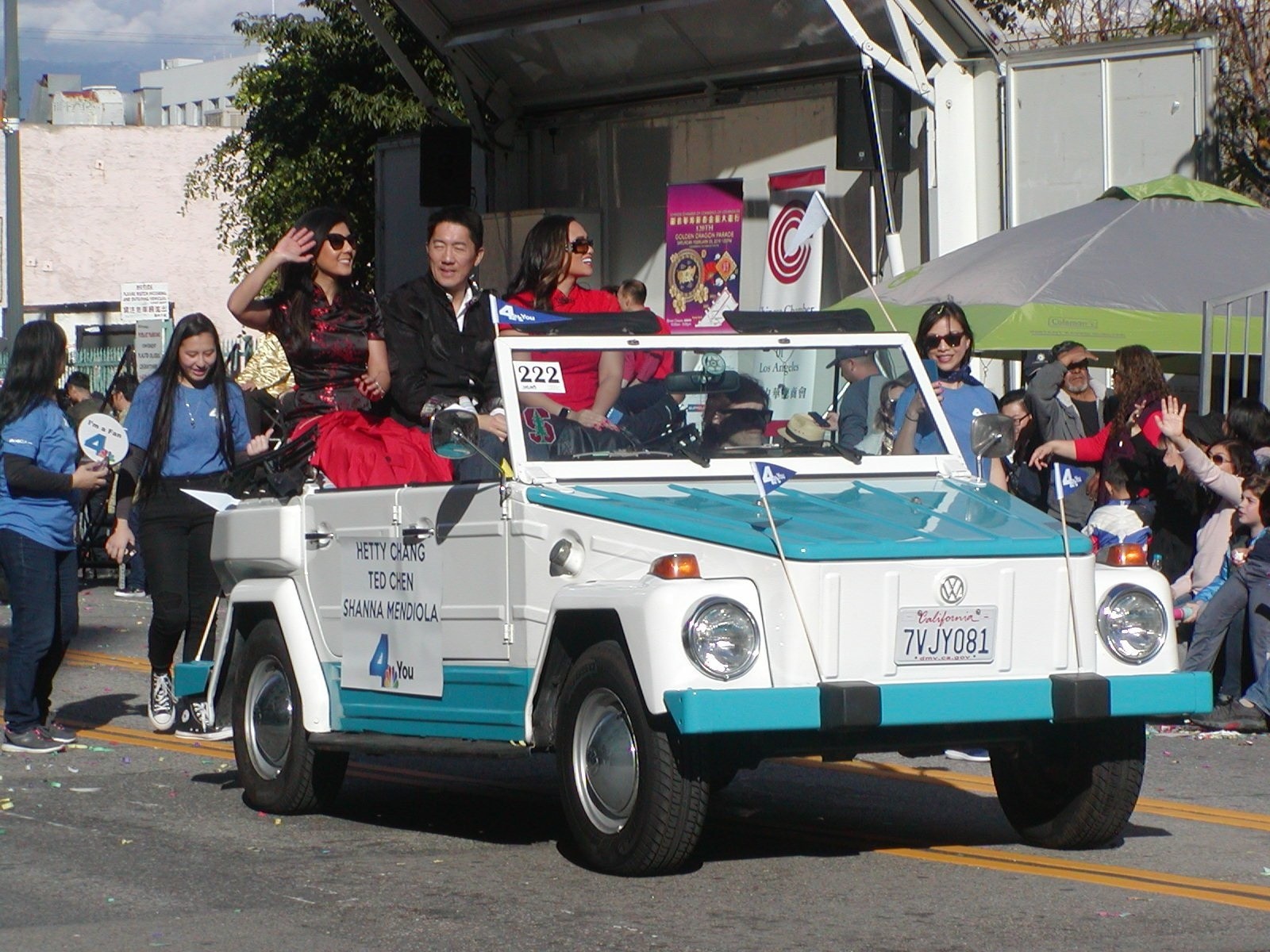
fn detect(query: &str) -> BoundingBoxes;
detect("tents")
[815,170,1269,361]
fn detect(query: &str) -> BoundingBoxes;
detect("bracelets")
[905,414,918,422]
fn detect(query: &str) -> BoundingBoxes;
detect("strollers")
[73,465,130,580]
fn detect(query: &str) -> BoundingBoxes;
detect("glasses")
[1013,413,1030,426]
[325,233,358,250]
[887,398,898,411]
[566,238,594,255]
[921,330,966,350]
[1111,366,1119,379]
[1204,452,1232,466]
[719,408,773,424]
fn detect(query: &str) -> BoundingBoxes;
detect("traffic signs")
[137,319,164,384]
[119,281,170,325]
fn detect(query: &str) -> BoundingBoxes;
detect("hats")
[1184,411,1226,446]
[826,346,876,368]
[777,413,825,448]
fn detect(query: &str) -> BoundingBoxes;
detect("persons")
[500,213,677,457]
[377,208,551,485]
[1000,389,1050,514]
[1028,342,1270,732]
[814,342,909,456]
[700,373,772,459]
[226,216,454,485]
[782,414,825,454]
[892,301,1009,493]
[0,311,299,754]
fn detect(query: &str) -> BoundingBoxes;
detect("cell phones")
[921,358,939,384]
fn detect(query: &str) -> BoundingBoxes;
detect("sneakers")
[174,696,234,741]
[148,668,176,730]
[39,711,77,743]
[1191,699,1268,732]
[2,724,65,753]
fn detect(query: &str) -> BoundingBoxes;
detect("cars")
[166,311,1213,878]
